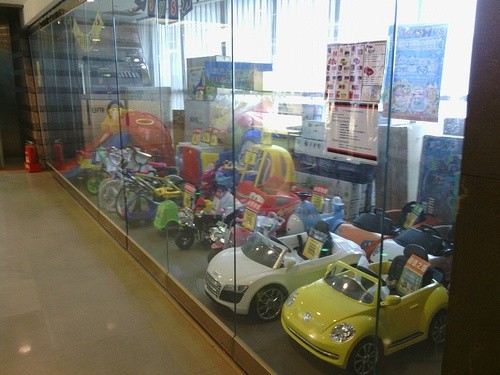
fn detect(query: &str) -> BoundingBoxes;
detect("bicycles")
[84,146,199,225]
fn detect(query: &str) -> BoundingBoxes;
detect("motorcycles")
[174,199,249,249]
[206,208,285,264]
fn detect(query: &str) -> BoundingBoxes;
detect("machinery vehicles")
[247,182,311,234]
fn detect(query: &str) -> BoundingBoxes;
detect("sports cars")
[281,243,451,375]
[367,224,454,286]
[333,201,442,260]
[204,220,367,321]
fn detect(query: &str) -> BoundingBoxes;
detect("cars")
[285,192,345,235]
